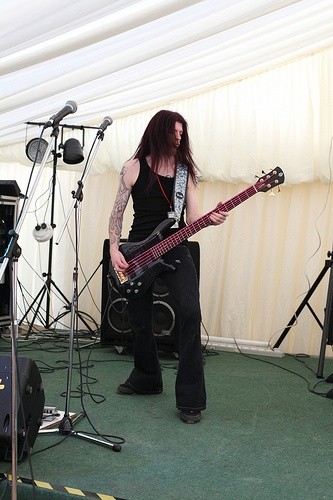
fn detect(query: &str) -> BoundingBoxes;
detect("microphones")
[44,101,78,130]
[97,117,112,136]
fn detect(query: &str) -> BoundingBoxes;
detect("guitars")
[108,166,285,304]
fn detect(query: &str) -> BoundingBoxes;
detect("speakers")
[100,238,201,357]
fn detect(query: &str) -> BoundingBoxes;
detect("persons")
[108,109,230,425]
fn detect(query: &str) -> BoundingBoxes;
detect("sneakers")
[180,410,201,424]
[118,384,163,394]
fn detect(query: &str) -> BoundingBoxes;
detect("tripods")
[39,128,123,453]
[274,242,333,380]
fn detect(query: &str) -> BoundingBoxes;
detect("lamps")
[26,137,50,163]
[61,124,86,164]
